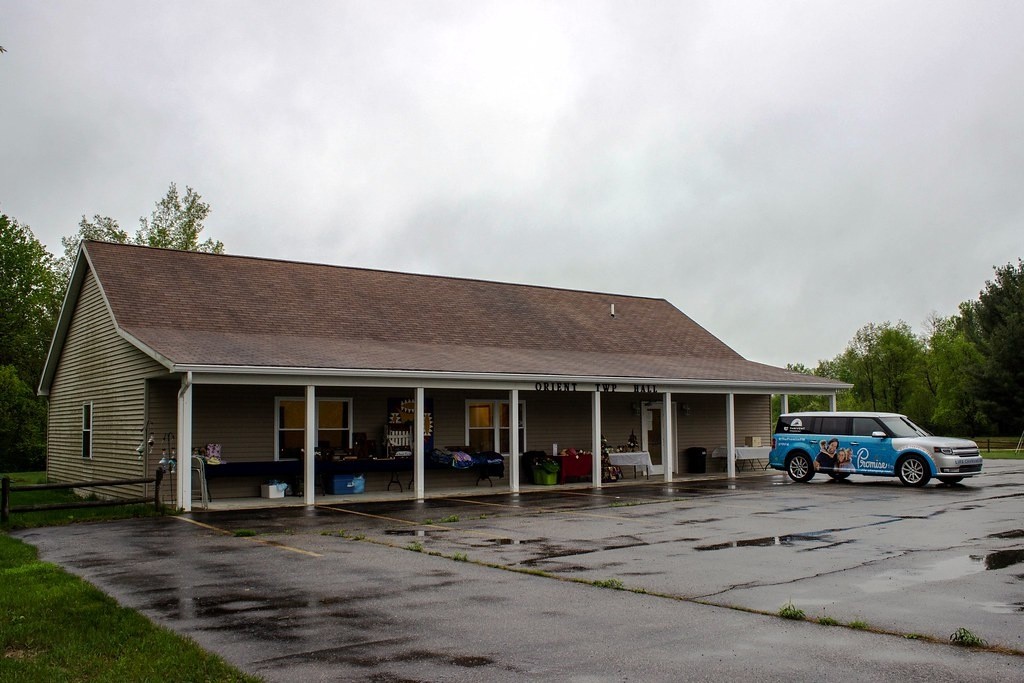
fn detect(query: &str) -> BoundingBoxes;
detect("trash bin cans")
[530,458,543,485]
[685,447,707,474]
[541,459,559,485]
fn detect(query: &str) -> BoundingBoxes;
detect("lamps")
[632,403,641,416]
[683,404,691,416]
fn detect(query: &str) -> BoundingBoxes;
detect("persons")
[814,439,856,469]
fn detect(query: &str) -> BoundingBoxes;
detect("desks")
[712,446,774,474]
[316,447,503,496]
[521,450,653,484]
[199,457,299,503]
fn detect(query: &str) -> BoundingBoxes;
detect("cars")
[768,412,983,489]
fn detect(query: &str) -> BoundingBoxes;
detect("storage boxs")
[261,483,286,499]
[744,436,762,447]
[319,473,365,496]
